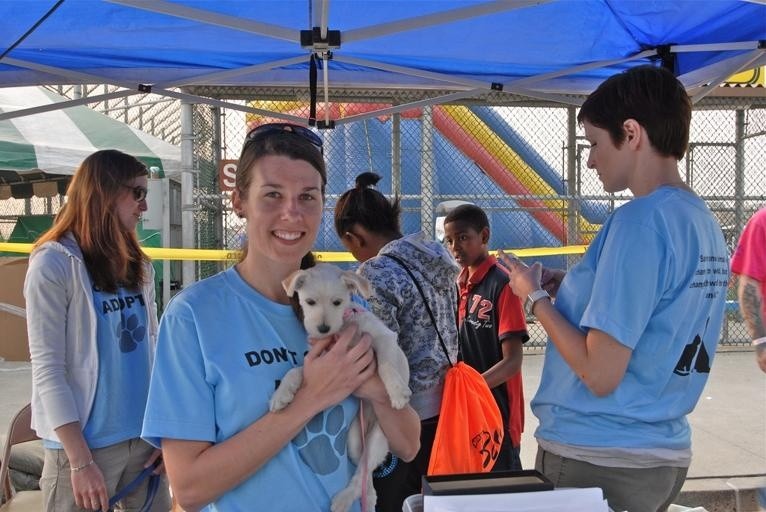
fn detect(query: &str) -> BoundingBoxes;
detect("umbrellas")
[0,87,214,202]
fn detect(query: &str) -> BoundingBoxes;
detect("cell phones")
[505,252,530,270]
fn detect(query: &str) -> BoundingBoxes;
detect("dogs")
[265,260,414,512]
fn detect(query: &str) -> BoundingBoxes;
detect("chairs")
[0,400,43,512]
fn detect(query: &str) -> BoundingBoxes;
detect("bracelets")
[751,336,766,347]
[69,458,96,475]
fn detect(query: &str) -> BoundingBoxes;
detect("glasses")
[244,123,323,153]
[124,185,146,201]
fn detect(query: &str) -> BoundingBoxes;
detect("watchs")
[521,287,552,316]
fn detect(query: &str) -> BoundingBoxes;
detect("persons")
[729,206,766,374]
[444,205,531,473]
[496,63,730,512]
[333,172,462,512]
[22,149,175,512]
[138,122,422,512]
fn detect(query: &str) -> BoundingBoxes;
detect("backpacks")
[383,246,504,476]
